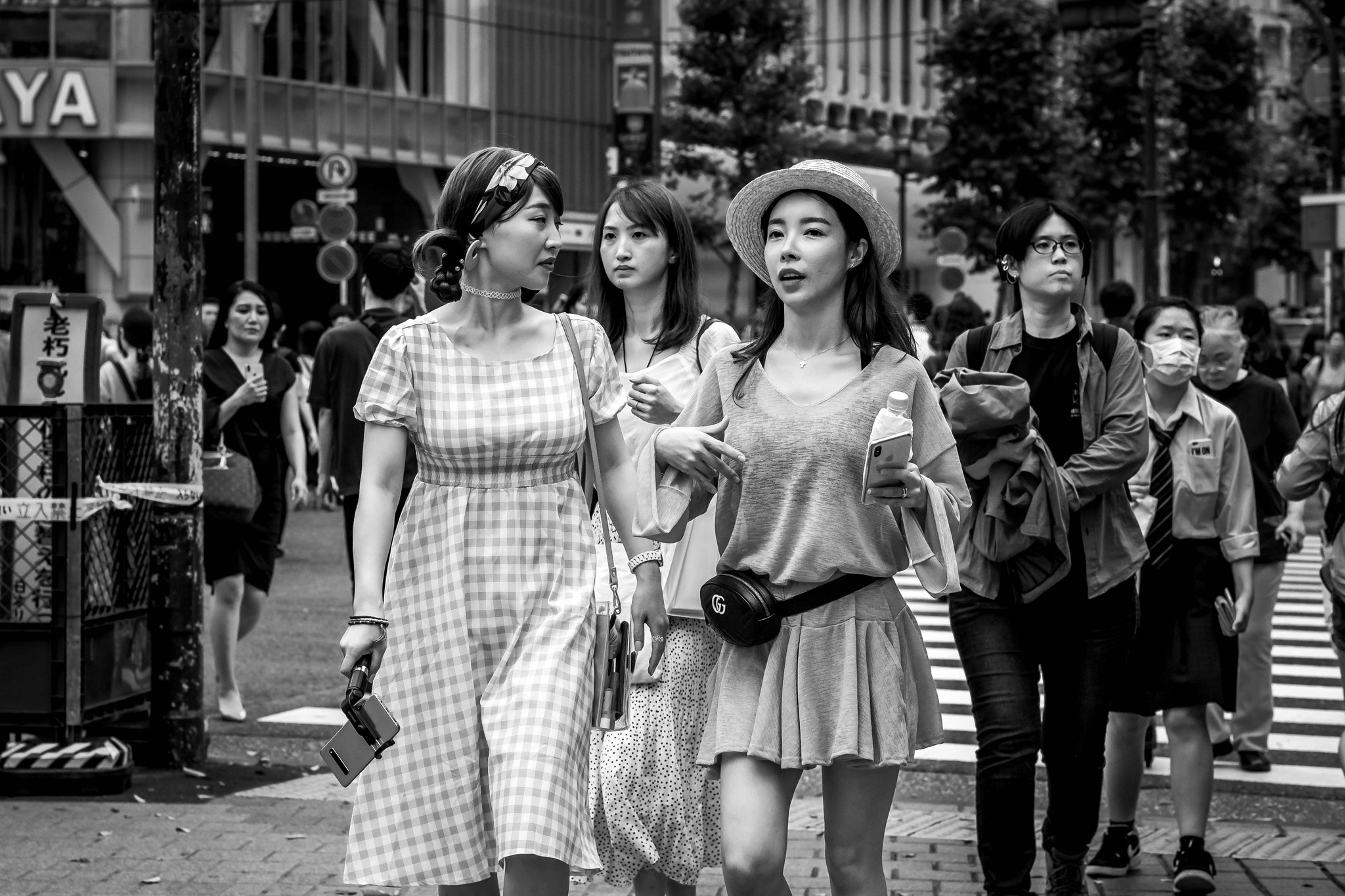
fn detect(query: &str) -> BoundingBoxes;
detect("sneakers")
[1174,846,1216,896]
[1084,825,1142,875]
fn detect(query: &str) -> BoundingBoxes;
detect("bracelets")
[348,616,390,644]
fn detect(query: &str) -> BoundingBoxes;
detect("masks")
[1142,338,1201,385]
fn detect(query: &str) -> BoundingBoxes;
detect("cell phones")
[861,432,913,505]
[320,694,400,788]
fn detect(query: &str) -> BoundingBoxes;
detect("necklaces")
[624,334,664,373]
[780,334,851,367]
[461,281,523,299]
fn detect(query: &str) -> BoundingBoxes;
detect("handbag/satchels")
[700,571,886,647]
[662,493,720,619]
[203,427,261,523]
[591,608,635,730]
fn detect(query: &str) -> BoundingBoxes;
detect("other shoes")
[1044,846,1091,896]
[1241,751,1269,772]
[1212,740,1233,758]
[1145,726,1158,767]
[217,687,246,721]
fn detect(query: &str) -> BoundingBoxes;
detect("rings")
[900,485,908,498]
[635,401,639,410]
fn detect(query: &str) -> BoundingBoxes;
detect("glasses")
[1026,239,1084,254]
[1199,349,1239,371]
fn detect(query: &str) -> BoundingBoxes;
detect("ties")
[1148,413,1188,568]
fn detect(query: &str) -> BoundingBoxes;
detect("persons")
[0,146,1345,896]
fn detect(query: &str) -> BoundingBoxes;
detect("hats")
[726,159,901,289]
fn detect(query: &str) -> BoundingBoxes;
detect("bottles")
[883,390,910,420]
